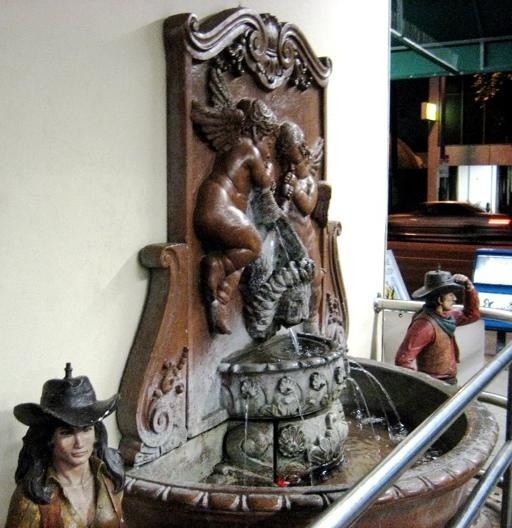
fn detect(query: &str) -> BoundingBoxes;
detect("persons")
[4,375,127,528]
[275,118,325,333]
[193,94,279,336]
[394,270,482,385]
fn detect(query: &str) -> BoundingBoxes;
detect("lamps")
[420,101,436,132]
